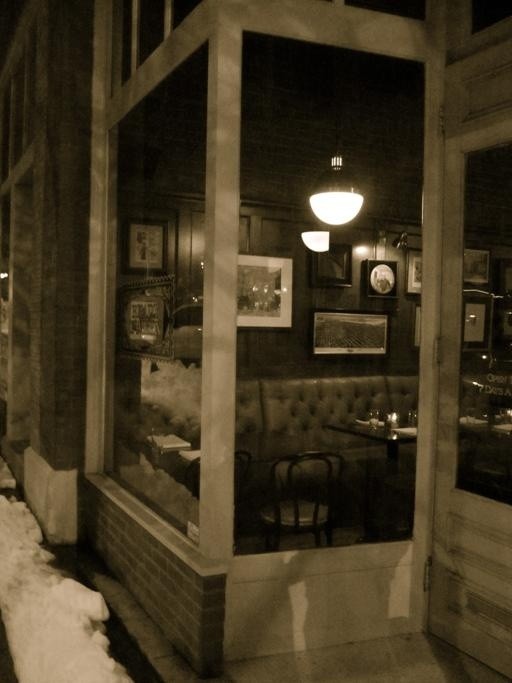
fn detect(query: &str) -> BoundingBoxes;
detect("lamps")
[307,118,364,226]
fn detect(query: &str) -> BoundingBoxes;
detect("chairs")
[254,450,345,553]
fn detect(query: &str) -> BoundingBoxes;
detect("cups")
[407,410,417,423]
[367,410,380,430]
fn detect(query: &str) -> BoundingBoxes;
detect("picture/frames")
[309,307,391,359]
[461,288,495,352]
[405,247,423,296]
[463,249,490,284]
[365,258,398,299]
[410,302,422,351]
[236,254,294,327]
[310,242,352,287]
[122,216,168,274]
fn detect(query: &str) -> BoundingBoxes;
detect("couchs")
[235,372,465,481]
